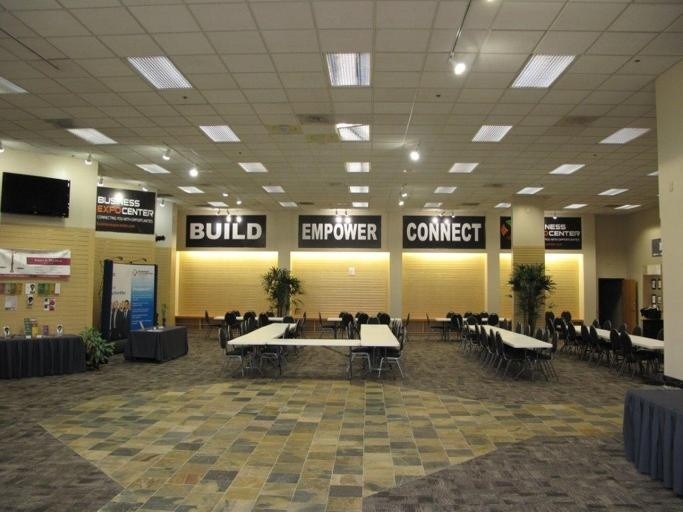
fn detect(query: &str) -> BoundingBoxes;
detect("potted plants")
[79,328,114,369]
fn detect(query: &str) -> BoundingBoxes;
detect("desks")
[627,389,682,499]
[129,326,187,364]
[1,334,86,378]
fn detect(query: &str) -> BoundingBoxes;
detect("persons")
[110,299,129,339]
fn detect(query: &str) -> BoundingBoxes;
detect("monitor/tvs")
[0,171,71,218]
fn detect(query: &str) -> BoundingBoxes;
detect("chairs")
[426,311,664,383]
[205,310,410,380]
